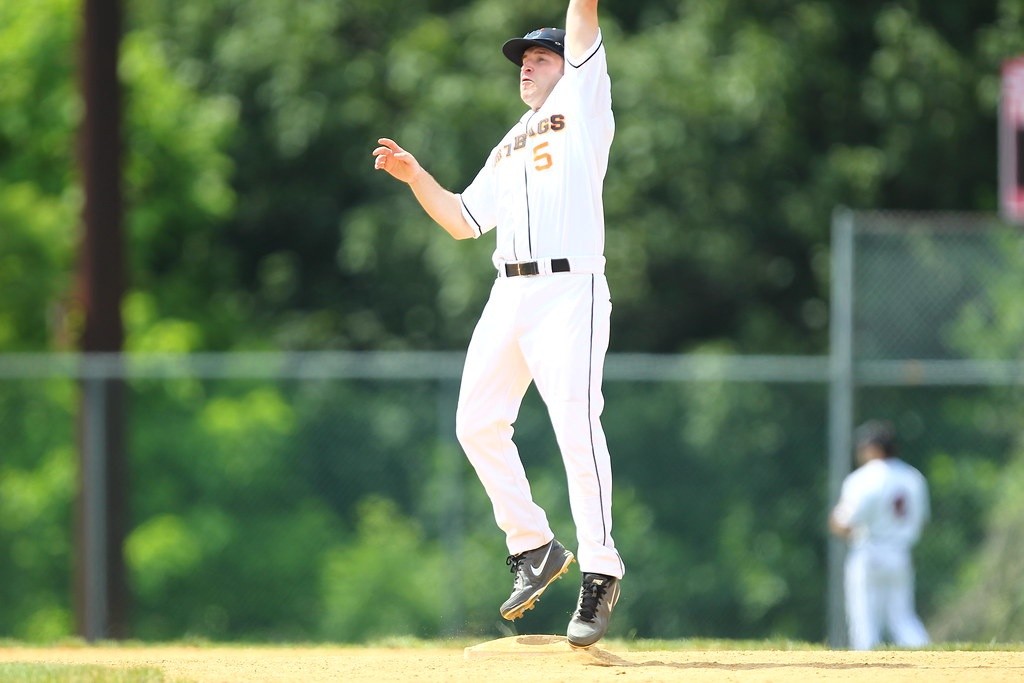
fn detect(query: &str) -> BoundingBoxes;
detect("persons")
[828,418,934,651]
[372,0,627,647]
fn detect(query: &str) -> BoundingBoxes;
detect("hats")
[502,28,565,68]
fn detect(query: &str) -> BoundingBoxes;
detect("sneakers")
[499,538,576,621]
[566,572,621,648]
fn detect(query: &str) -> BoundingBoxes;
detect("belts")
[505,259,570,277]
